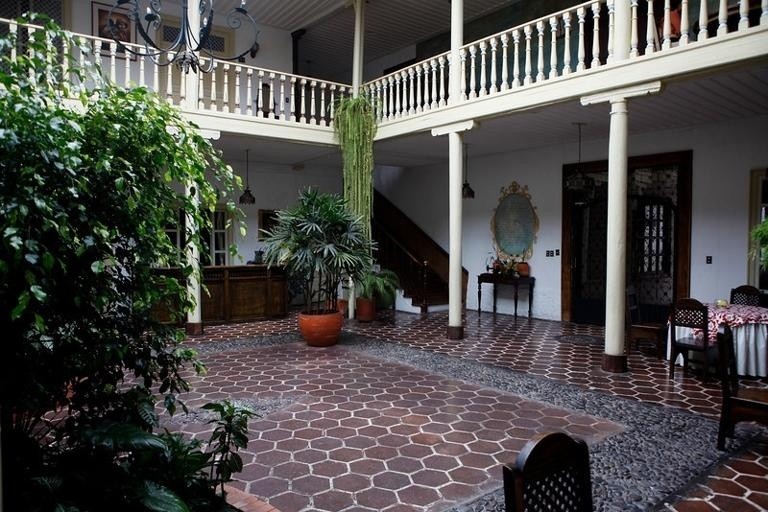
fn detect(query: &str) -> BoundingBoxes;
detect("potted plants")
[339,261,402,321]
[516,248,529,276]
[260,185,379,346]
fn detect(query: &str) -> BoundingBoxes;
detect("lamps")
[239,150,255,204]
[462,142,475,199]
[107,1,261,74]
[567,123,593,191]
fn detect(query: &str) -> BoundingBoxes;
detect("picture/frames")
[92,1,137,61]
[258,209,282,241]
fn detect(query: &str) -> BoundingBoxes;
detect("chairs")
[669,285,764,387]
[717,324,768,451]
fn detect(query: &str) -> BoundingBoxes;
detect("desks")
[478,273,535,320]
[666,304,768,378]
